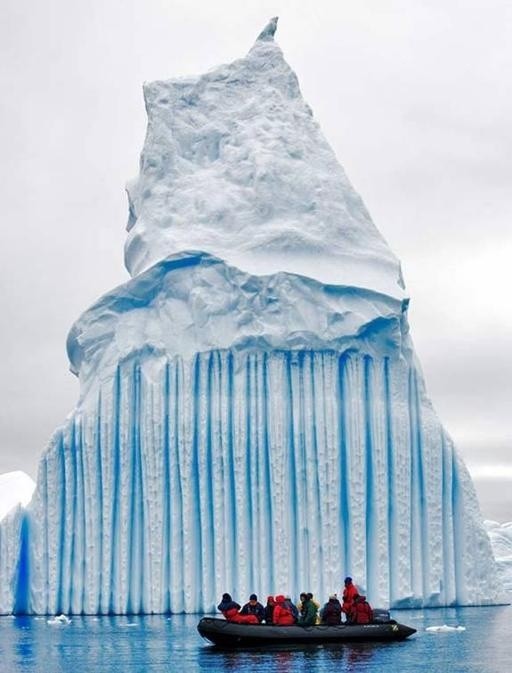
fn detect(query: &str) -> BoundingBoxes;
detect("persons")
[341,576,360,625]
[239,593,266,625]
[264,591,372,625]
[218,593,259,625]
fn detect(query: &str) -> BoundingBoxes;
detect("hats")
[222,577,366,603]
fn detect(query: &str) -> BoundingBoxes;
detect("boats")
[197,641,415,671]
[196,614,417,646]
